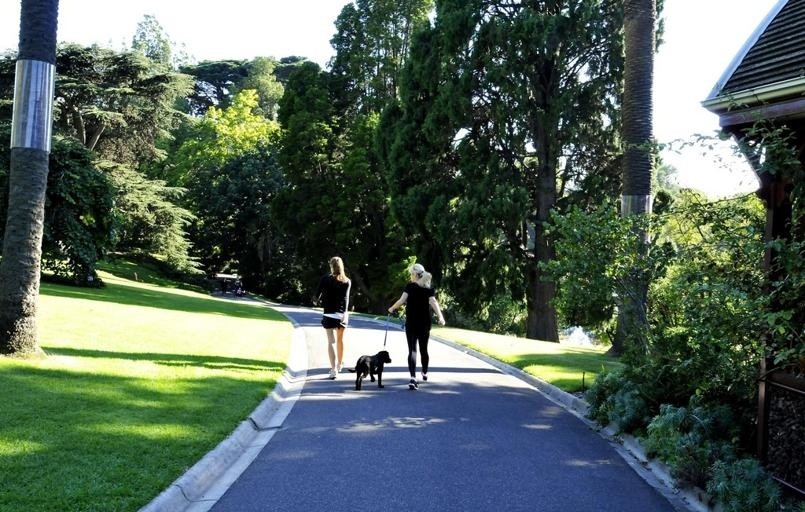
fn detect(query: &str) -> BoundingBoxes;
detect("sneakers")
[330,361,344,379]
[409,379,419,390]
[421,369,428,381]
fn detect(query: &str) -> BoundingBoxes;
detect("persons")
[315,256,351,379]
[388,263,445,390]
[233,277,245,297]
[221,278,227,294]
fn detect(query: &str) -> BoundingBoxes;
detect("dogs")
[347,351,391,391]
